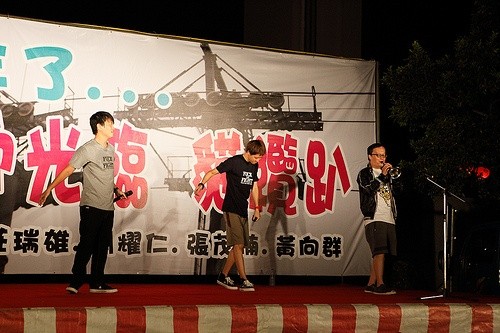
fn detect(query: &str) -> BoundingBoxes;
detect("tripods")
[417,177,479,302]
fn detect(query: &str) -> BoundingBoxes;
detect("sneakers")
[217,272,238,290]
[239,278,255,292]
[89,284,119,294]
[65,285,79,294]
[364,283,397,295]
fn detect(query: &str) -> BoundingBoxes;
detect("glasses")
[371,154,387,158]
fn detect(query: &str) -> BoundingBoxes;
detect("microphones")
[114,191,133,202]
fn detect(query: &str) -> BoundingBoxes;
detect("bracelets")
[114,186,119,194]
[198,183,204,190]
[255,206,260,210]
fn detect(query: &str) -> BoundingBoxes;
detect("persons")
[39,111,127,293]
[357,143,397,294]
[194,140,266,291]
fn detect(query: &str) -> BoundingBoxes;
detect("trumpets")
[382,163,403,179]
